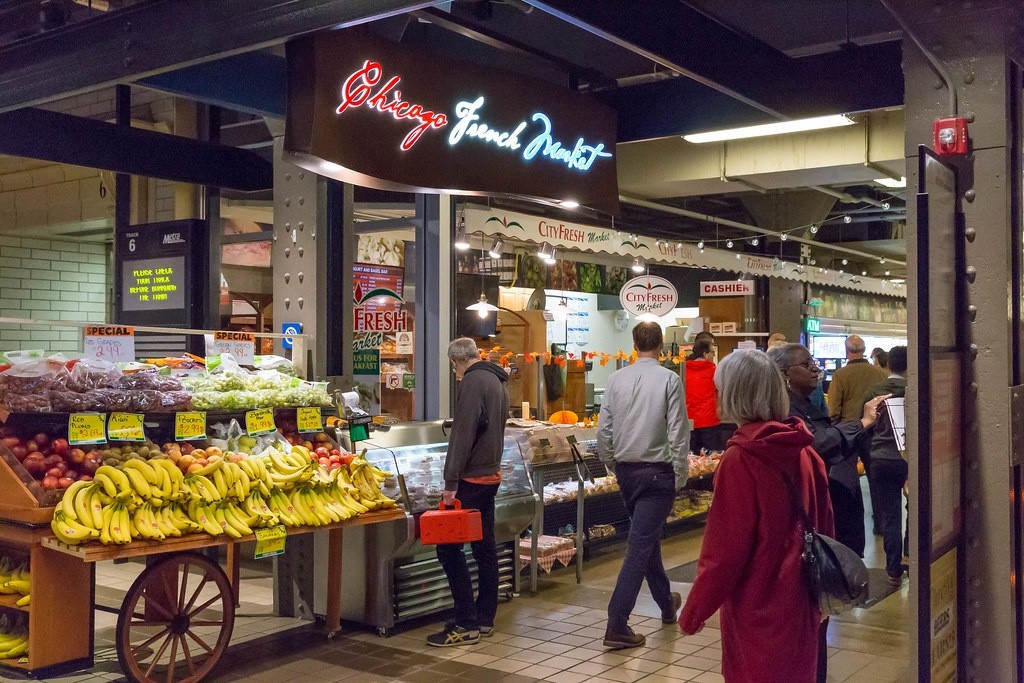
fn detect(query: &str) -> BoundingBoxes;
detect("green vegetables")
[580,264,602,292]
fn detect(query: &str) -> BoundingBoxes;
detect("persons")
[685,332,907,585]
[426,337,511,647]
[598,321,691,647]
[677,350,836,683]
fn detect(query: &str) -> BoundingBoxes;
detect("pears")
[94,440,163,468]
[521,255,547,288]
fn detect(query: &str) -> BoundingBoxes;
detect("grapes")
[0,370,334,413]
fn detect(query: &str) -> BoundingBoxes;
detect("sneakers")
[602,627,646,648]
[478,624,495,636]
[427,620,481,647]
[662,592,681,623]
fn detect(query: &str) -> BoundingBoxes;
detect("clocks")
[615,309,629,331]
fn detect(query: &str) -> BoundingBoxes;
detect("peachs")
[0,423,101,504]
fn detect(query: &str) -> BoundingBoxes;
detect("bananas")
[50,444,397,546]
[0,554,31,607]
[0,623,29,659]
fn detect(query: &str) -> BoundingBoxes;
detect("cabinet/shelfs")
[521,453,595,596]
[1,526,95,680]
[664,472,718,531]
[0,406,340,523]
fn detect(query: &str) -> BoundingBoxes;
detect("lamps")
[466,234,499,320]
[539,241,556,265]
[457,221,472,251]
[680,109,861,144]
[631,256,645,270]
[489,235,505,259]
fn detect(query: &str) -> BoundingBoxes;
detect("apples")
[162,420,350,475]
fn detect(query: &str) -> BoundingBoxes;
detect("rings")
[873,398,877,401]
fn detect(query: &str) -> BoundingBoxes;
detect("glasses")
[786,360,817,370]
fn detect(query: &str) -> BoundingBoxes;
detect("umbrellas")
[441,417,488,436]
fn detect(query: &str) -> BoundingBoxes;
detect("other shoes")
[889,564,909,585]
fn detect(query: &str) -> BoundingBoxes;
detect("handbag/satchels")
[804,532,869,617]
